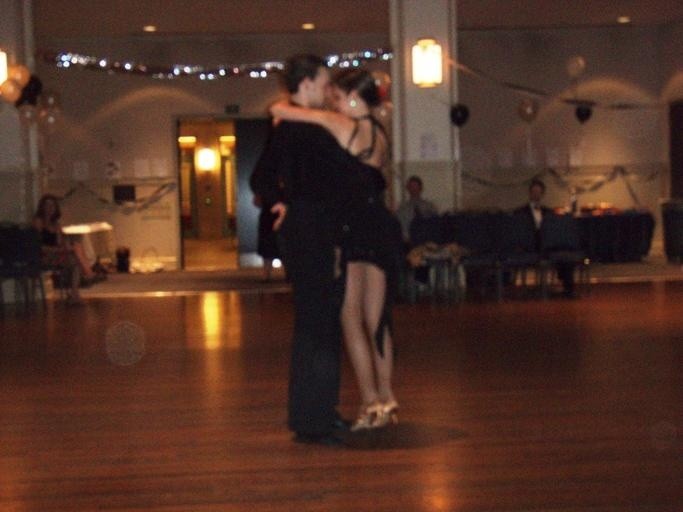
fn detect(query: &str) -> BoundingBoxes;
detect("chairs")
[399,210,594,302]
[0,219,72,312]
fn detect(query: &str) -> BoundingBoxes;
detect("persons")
[268,69,400,434]
[392,171,438,285]
[246,53,391,443]
[498,180,578,298]
[32,195,102,303]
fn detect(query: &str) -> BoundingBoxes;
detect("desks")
[63,222,114,284]
[556,200,655,267]
[656,195,683,266]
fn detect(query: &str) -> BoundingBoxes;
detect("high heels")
[350,401,400,432]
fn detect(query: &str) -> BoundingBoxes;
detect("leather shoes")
[293,419,349,447]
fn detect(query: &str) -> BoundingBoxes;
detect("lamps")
[0,45,42,110]
[192,146,218,190]
[410,37,443,90]
